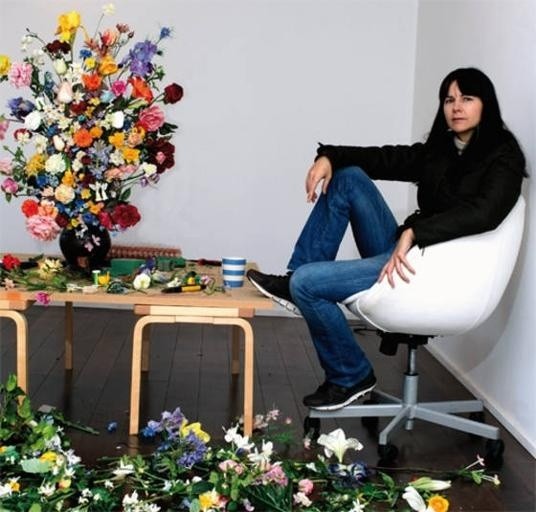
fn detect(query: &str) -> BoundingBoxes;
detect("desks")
[1,263,272,442]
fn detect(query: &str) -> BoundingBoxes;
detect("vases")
[54,230,122,281]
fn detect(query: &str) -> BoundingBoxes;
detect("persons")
[248,67,527,412]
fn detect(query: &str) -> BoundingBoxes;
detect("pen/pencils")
[162,285,206,293]
[189,259,222,266]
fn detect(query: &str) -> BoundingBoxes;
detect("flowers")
[1,2,217,243]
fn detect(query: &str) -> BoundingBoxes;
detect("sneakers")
[302,369,377,411]
[247,270,302,315]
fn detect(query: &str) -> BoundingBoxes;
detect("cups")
[222,257,248,287]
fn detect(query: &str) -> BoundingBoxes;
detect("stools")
[300,195,526,469]
